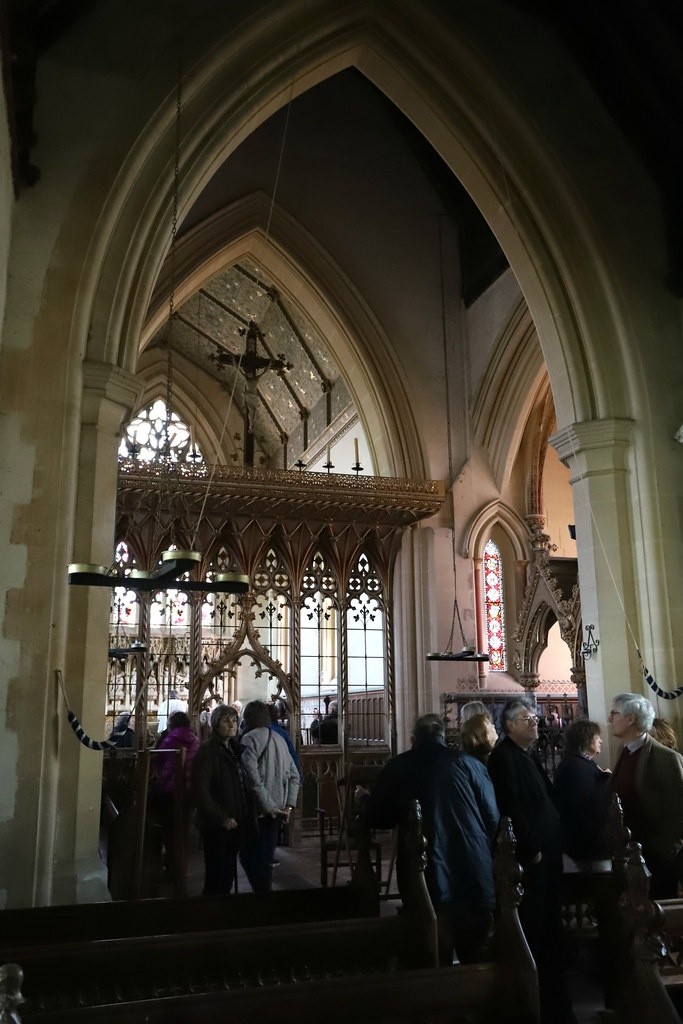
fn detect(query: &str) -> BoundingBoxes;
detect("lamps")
[425,213,490,661]
[64,2,249,592]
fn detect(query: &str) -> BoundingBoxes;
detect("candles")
[190,425,195,451]
[134,431,136,444]
[355,438,359,463]
[327,445,330,463]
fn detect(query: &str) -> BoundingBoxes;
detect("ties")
[619,746,629,798]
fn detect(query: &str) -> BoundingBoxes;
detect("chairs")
[318,784,382,888]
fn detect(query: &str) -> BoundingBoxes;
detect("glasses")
[611,710,621,715]
[515,716,539,725]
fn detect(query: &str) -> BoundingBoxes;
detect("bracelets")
[285,807,292,811]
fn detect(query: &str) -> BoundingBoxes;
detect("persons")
[310,700,338,744]
[95,698,301,897]
[355,693,683,1024]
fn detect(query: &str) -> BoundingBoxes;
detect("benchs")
[0,789,683,1024]
